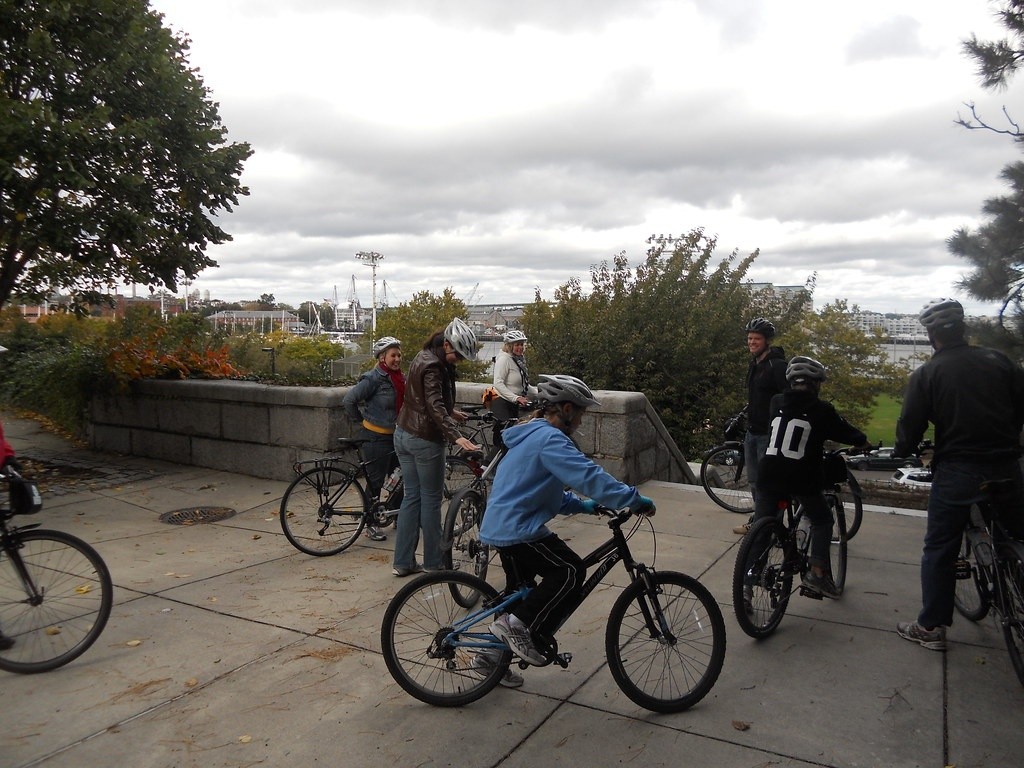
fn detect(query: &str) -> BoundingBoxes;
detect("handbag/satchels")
[482,387,499,412]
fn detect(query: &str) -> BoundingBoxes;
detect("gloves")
[630,494,656,517]
[581,498,600,515]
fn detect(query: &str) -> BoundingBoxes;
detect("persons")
[733,317,790,534]
[742,355,873,614]
[468,375,656,687]
[473,330,538,483]
[342,336,407,540]
[891,297,1024,652]
[393,317,482,576]
[0,347,23,649]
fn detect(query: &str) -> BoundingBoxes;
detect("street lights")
[355,250,385,356]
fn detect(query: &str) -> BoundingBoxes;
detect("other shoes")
[0,632,14,650]
[421,561,460,572]
[392,562,423,575]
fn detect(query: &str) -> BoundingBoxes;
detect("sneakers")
[800,571,843,600]
[733,523,752,534]
[743,588,754,615]
[470,650,523,687]
[487,612,545,665]
[364,526,387,541]
[896,620,946,650]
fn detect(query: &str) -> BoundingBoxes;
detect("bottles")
[379,466,403,499]
[796,514,811,549]
[966,523,996,596]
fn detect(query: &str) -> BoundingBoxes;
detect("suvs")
[890,468,933,492]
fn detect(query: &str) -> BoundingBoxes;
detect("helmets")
[785,355,827,382]
[444,316,479,362]
[745,317,775,338]
[918,298,964,328]
[373,337,400,359]
[535,373,601,407]
[504,330,528,343]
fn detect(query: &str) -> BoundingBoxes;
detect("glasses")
[449,344,465,361]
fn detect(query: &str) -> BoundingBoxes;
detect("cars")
[845,447,924,472]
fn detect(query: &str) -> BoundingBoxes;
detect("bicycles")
[0,465,114,675]
[914,438,1024,691]
[277,438,488,557]
[442,412,521,610]
[731,439,879,642]
[437,397,582,499]
[699,401,862,542]
[379,502,729,714]
